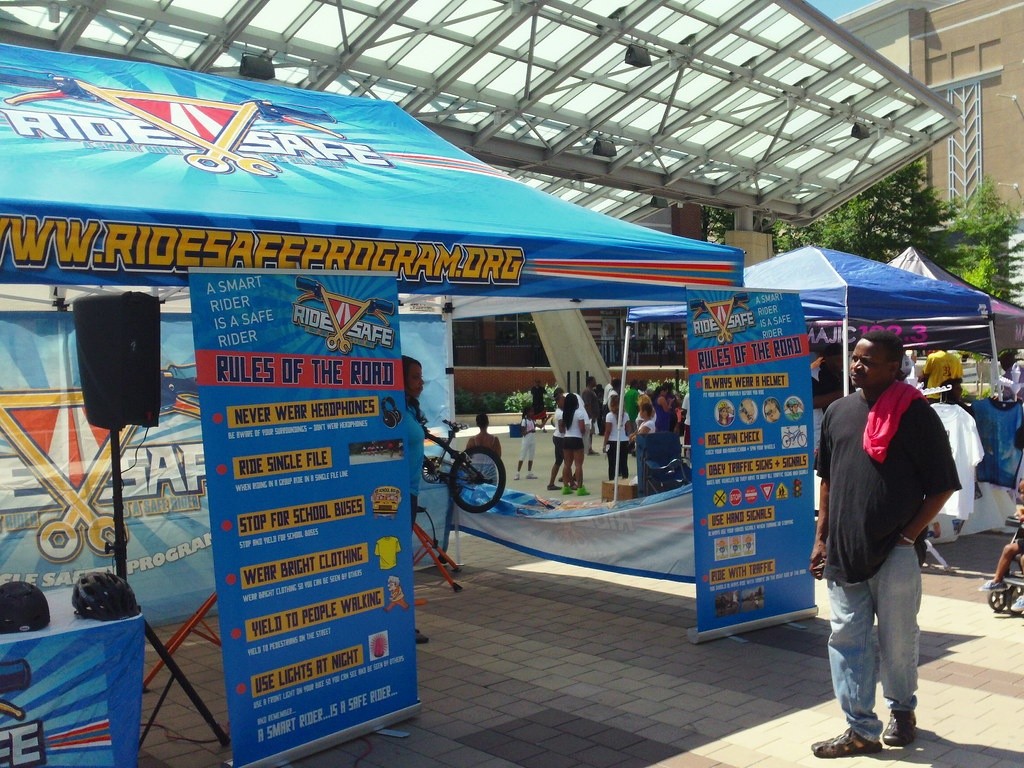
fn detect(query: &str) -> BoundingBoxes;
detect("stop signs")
[745,486,757,504]
[730,489,742,505]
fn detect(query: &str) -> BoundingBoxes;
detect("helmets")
[0,581,49,634]
[72,572,139,620]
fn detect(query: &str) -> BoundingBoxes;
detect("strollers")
[987,516,1024,614]
[530,407,551,433]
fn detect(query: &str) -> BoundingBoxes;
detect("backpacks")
[509,419,535,438]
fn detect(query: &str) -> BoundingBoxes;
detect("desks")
[0,588,231,768]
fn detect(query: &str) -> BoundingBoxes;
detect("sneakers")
[979,579,1007,591]
[1011,594,1024,612]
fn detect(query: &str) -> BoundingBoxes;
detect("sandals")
[882,710,916,746]
[811,727,882,757]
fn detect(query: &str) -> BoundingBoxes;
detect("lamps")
[239,51,274,79]
[625,45,652,67]
[593,137,617,158]
[650,196,669,210]
[851,123,871,140]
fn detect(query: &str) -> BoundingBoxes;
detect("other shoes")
[586,450,599,455]
[526,474,537,479]
[563,485,573,494]
[576,487,590,495]
[514,473,519,480]
[416,632,429,643]
[547,484,561,490]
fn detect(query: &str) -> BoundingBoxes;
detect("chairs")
[638,431,693,497]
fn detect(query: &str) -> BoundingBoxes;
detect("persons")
[919,350,1024,611]
[466,414,501,487]
[515,377,690,496]
[403,355,429,645]
[808,331,963,757]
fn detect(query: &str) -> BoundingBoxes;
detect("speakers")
[74,292,161,431]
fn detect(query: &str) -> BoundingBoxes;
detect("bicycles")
[422,419,506,513]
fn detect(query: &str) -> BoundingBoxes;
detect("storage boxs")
[602,478,637,503]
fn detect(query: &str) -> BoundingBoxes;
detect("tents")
[0,43,744,620]
[612,244,1003,501]
[805,245,1024,397]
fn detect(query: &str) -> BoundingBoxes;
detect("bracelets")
[900,532,914,544]
